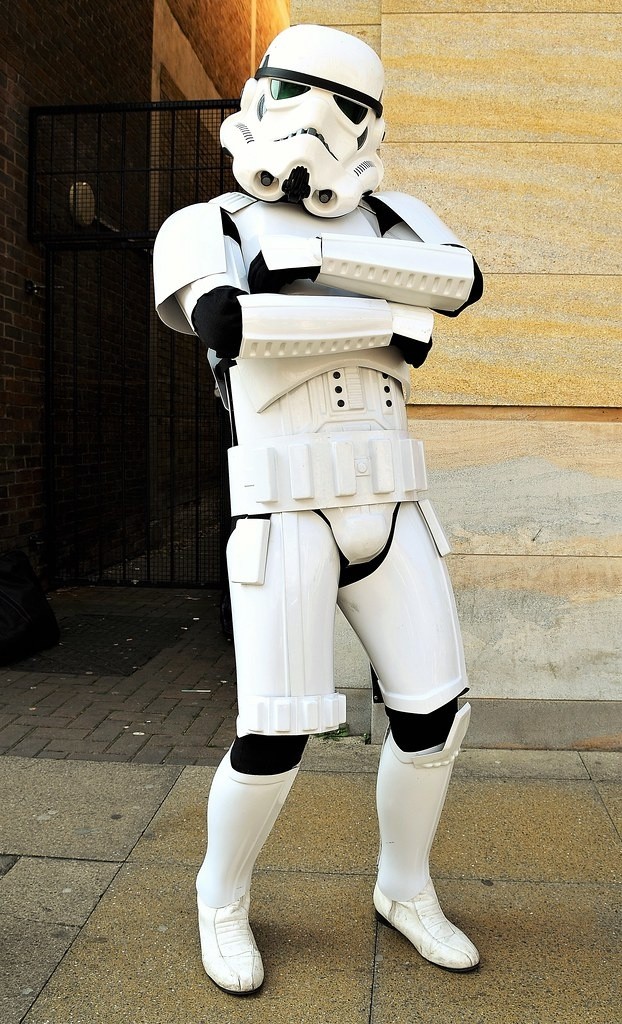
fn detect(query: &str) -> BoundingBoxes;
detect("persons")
[152,22,486,995]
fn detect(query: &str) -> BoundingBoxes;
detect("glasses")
[269,77,370,125]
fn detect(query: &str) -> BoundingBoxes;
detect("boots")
[373,701,481,974]
[196,741,302,996]
